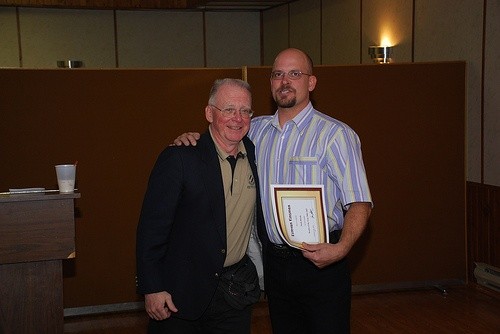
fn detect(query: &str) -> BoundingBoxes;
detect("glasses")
[269,71,311,80]
[212,104,255,119]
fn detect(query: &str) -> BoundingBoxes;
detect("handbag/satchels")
[211,254,261,309]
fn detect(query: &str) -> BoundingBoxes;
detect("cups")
[55,165,76,194]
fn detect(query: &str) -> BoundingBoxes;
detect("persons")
[134,78,266,334]
[171,47,374,334]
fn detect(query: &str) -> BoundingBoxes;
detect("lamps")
[367,46,393,64]
